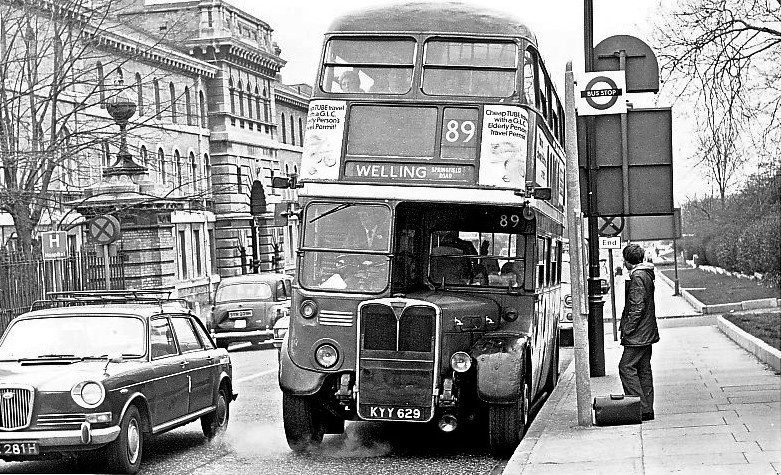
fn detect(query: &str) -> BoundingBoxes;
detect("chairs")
[428,237,473,287]
[147,340,180,354]
[500,260,526,285]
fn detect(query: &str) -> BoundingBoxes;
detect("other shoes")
[642,411,655,421]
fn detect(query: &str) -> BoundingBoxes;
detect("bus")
[273,0,566,460]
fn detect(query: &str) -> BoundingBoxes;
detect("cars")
[205,273,294,350]
[560,257,611,331]
[0,288,238,475]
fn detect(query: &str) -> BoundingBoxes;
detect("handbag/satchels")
[593,394,643,426]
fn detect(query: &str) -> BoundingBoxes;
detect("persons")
[436,230,490,264]
[335,207,389,271]
[501,262,520,288]
[617,244,661,421]
[377,67,409,94]
[339,71,366,93]
[470,264,488,285]
